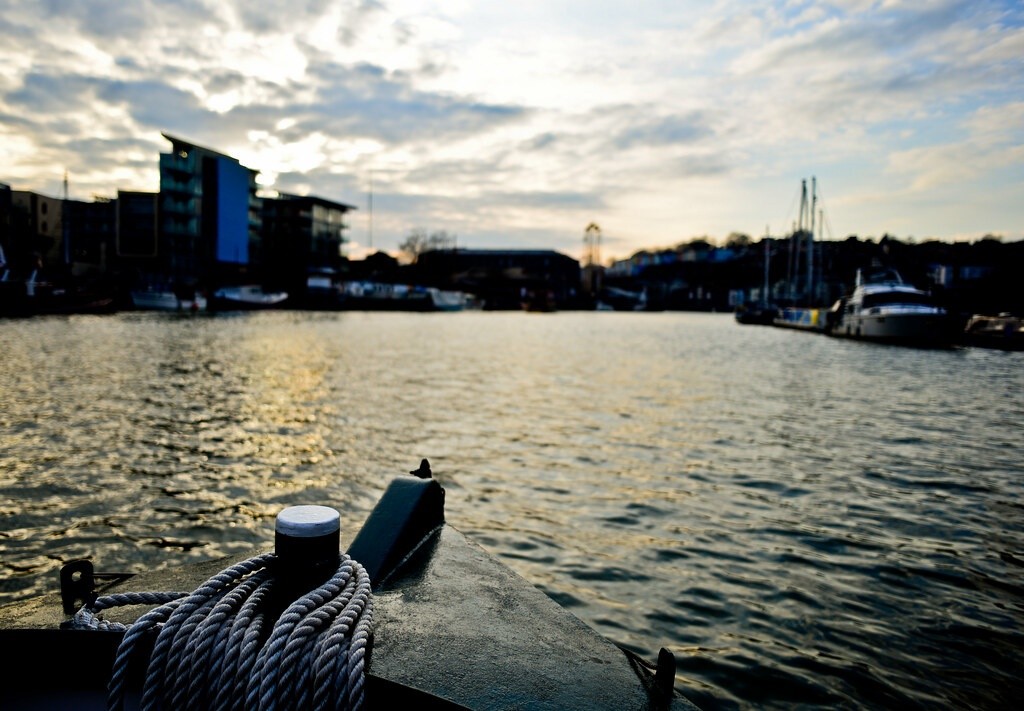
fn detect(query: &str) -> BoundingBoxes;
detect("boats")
[829,265,967,345]
[0,259,293,317]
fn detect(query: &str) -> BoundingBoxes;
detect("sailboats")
[735,176,839,332]
